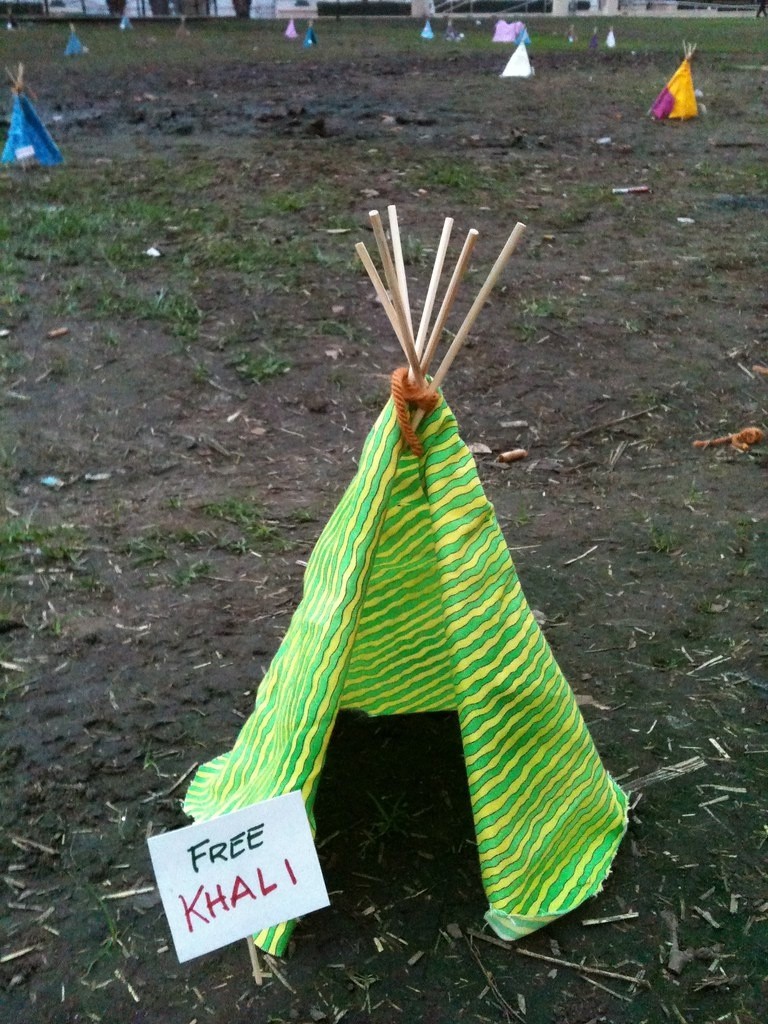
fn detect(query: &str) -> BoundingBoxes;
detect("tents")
[120,17,133,29]
[178,366,632,958]
[64,31,89,54]
[499,40,533,77]
[286,20,319,47]
[6,89,67,167]
[648,57,699,121]
[421,22,455,41]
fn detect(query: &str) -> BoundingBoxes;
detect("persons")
[756,0,768,17]
